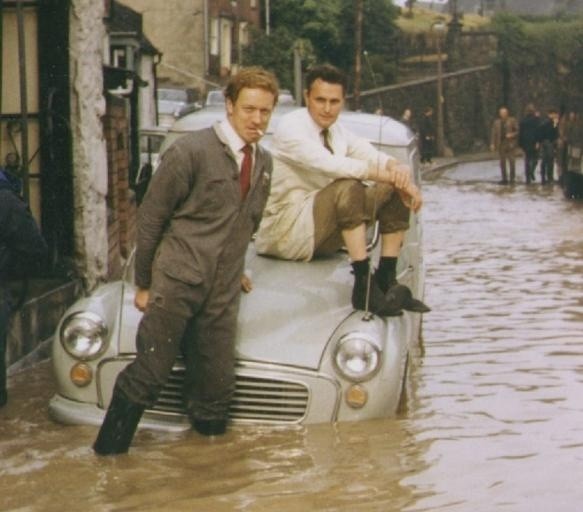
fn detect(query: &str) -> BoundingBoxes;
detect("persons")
[378,104,436,166]
[253,64,432,316]
[489,101,575,184]
[129,163,152,209]
[0,187,90,408]
[91,63,280,457]
[0,152,23,196]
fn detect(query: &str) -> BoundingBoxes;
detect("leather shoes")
[352,273,397,317]
[374,268,431,314]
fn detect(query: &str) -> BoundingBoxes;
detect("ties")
[319,128,333,155]
[235,144,256,201]
